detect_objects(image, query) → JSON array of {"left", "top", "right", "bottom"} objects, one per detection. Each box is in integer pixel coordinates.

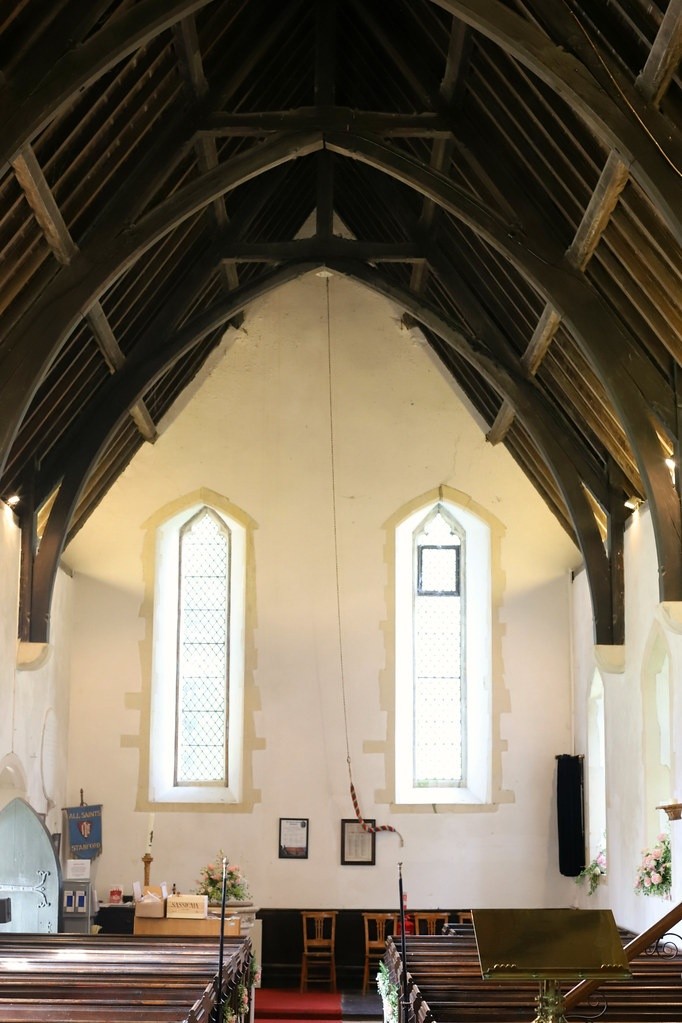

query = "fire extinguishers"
[{"left": 396, "top": 912, "right": 415, "bottom": 935}]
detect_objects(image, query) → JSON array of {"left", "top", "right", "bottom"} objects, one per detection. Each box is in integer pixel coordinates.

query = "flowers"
[
  {"left": 246, "top": 949, "right": 261, "bottom": 988},
  {"left": 234, "top": 982, "right": 250, "bottom": 1012},
  {"left": 189, "top": 849, "right": 253, "bottom": 903},
  {"left": 633, "top": 833, "right": 671, "bottom": 902},
  {"left": 574, "top": 830, "right": 607, "bottom": 897},
  {"left": 375, "top": 960, "right": 399, "bottom": 1023},
  {"left": 221, "top": 993, "right": 238, "bottom": 1023}
]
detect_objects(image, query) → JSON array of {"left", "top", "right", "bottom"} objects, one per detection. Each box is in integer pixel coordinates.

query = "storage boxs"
[
  {"left": 166, "top": 894, "right": 208, "bottom": 919},
  {"left": 132, "top": 881, "right": 171, "bottom": 918}
]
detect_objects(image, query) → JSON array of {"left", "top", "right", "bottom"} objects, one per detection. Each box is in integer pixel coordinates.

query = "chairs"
[
  {"left": 413, "top": 913, "right": 450, "bottom": 935},
  {"left": 362, "top": 913, "right": 401, "bottom": 997},
  {"left": 300, "top": 911, "right": 338, "bottom": 996}
]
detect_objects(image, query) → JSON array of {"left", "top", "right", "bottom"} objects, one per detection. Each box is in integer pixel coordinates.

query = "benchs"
[
  {"left": 382, "top": 930, "right": 682, "bottom": 1023},
  {"left": 0, "top": 933, "right": 252, "bottom": 1023}
]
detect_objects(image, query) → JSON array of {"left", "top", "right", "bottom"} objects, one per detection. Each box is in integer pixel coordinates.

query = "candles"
[{"left": 145, "top": 813, "right": 155, "bottom": 854}]
